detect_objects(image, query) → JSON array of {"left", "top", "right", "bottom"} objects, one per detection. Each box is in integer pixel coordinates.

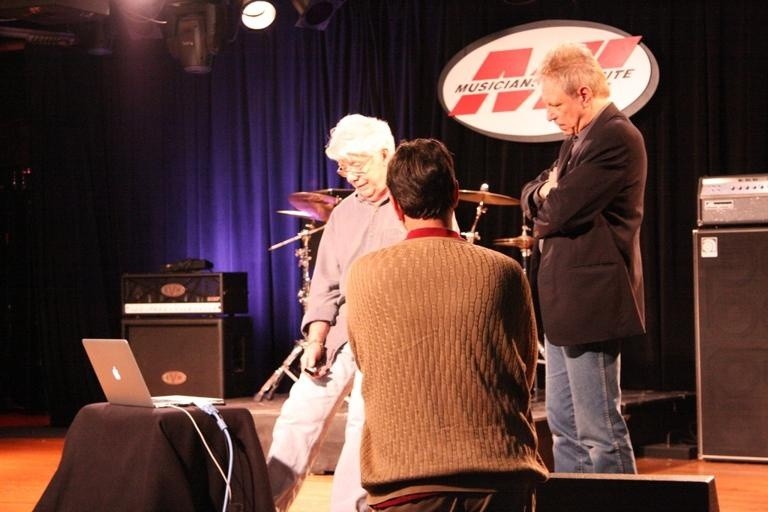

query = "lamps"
[
  {"left": 238, "top": 0, "right": 278, "bottom": 31},
  {"left": 176, "top": 15, "right": 214, "bottom": 76}
]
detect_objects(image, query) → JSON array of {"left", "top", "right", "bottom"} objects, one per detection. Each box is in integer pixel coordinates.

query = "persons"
[
  {"left": 266, "top": 114, "right": 460, "bottom": 511},
  {"left": 344, "top": 138, "right": 550, "bottom": 512},
  {"left": 519, "top": 44, "right": 647, "bottom": 475}
]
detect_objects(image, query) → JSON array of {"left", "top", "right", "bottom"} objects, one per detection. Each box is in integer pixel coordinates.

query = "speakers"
[
  {"left": 691, "top": 225, "right": 768, "bottom": 463},
  {"left": 120, "top": 315, "right": 254, "bottom": 398}
]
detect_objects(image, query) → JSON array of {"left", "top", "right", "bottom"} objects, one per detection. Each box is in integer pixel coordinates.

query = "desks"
[{"left": 30, "top": 403, "right": 277, "bottom": 511}]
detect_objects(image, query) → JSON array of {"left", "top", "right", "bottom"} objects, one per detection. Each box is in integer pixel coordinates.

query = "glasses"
[{"left": 336, "top": 158, "right": 371, "bottom": 177}]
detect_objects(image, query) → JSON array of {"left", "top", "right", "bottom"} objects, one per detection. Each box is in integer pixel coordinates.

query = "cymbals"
[
  {"left": 277, "top": 210, "right": 315, "bottom": 220},
  {"left": 458, "top": 189, "right": 520, "bottom": 206},
  {"left": 288, "top": 192, "right": 343, "bottom": 223},
  {"left": 315, "top": 189, "right": 355, "bottom": 194},
  {"left": 268, "top": 226, "right": 326, "bottom": 250},
  {"left": 494, "top": 236, "right": 537, "bottom": 249}
]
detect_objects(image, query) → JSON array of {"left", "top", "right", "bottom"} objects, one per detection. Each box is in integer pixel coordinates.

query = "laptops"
[{"left": 82, "top": 338, "right": 225, "bottom": 408}]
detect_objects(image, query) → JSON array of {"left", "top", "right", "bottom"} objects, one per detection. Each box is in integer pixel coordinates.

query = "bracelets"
[{"left": 303, "top": 338, "right": 325, "bottom": 347}]
